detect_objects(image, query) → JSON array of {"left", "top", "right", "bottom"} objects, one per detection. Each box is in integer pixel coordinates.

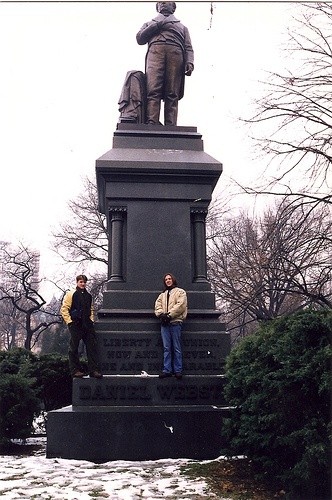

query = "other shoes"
[
  {"left": 159, "top": 373, "right": 172, "bottom": 378},
  {"left": 92, "top": 368, "right": 103, "bottom": 378},
  {"left": 175, "top": 372, "right": 182, "bottom": 379},
  {"left": 71, "top": 370, "right": 85, "bottom": 378}
]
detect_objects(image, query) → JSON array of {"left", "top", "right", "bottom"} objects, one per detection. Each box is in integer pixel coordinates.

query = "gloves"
[{"left": 159, "top": 312, "right": 172, "bottom": 328}]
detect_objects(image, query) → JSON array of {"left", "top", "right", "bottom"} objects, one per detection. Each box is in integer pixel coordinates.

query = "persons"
[
  {"left": 60, "top": 275, "right": 103, "bottom": 379},
  {"left": 136, "top": 2, "right": 195, "bottom": 126},
  {"left": 154, "top": 273, "right": 188, "bottom": 379}
]
